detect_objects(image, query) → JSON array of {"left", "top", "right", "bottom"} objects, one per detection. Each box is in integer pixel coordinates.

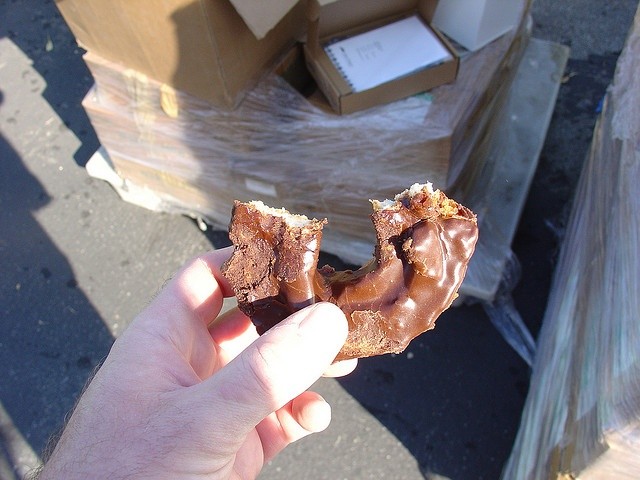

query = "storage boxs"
[
  {"left": 432, "top": 1, "right": 526, "bottom": 53},
  {"left": 51, "top": 0, "right": 314, "bottom": 113},
  {"left": 301, "top": 0, "right": 463, "bottom": 118}
]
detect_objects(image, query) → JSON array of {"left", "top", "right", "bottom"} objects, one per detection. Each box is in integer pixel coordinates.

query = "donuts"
[{"left": 222, "top": 181, "right": 479, "bottom": 364}]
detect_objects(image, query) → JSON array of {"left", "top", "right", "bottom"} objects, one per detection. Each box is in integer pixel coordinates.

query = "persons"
[{"left": 24, "top": 243, "right": 361, "bottom": 480}]
